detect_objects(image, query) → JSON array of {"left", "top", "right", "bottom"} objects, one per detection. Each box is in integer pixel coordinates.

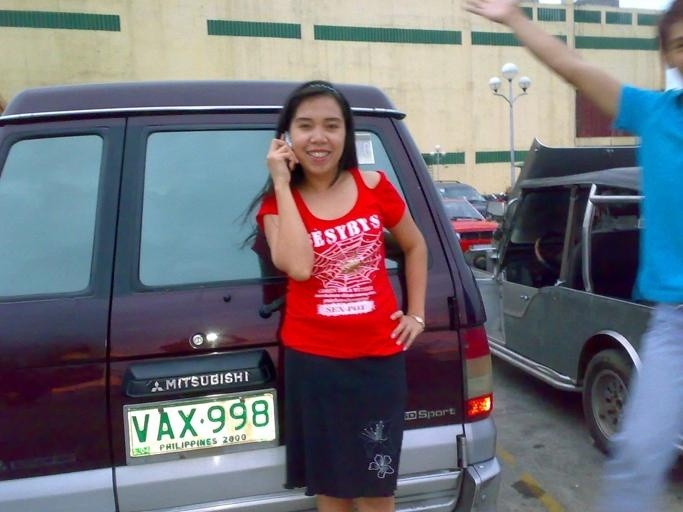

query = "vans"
[{"left": 1, "top": 79, "right": 501, "bottom": 512}]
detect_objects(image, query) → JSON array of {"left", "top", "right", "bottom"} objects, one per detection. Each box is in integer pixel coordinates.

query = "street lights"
[{"left": 491, "top": 62, "right": 531, "bottom": 184}]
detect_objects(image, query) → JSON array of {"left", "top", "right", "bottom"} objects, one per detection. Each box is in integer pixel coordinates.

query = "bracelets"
[{"left": 405, "top": 314, "right": 427, "bottom": 330}]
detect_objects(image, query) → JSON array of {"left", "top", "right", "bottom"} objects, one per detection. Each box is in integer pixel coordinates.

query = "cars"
[
  {"left": 433, "top": 179, "right": 500, "bottom": 264},
  {"left": 464, "top": 139, "right": 683, "bottom": 458}
]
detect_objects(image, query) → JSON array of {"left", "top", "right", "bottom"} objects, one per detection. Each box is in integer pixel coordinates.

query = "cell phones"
[{"left": 284, "top": 131, "right": 293, "bottom": 148}]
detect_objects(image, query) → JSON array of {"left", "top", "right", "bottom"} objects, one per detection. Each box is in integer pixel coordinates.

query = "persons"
[
  {"left": 459, "top": 0, "right": 682, "bottom": 512},
  {"left": 234, "top": 80, "right": 430, "bottom": 511}
]
